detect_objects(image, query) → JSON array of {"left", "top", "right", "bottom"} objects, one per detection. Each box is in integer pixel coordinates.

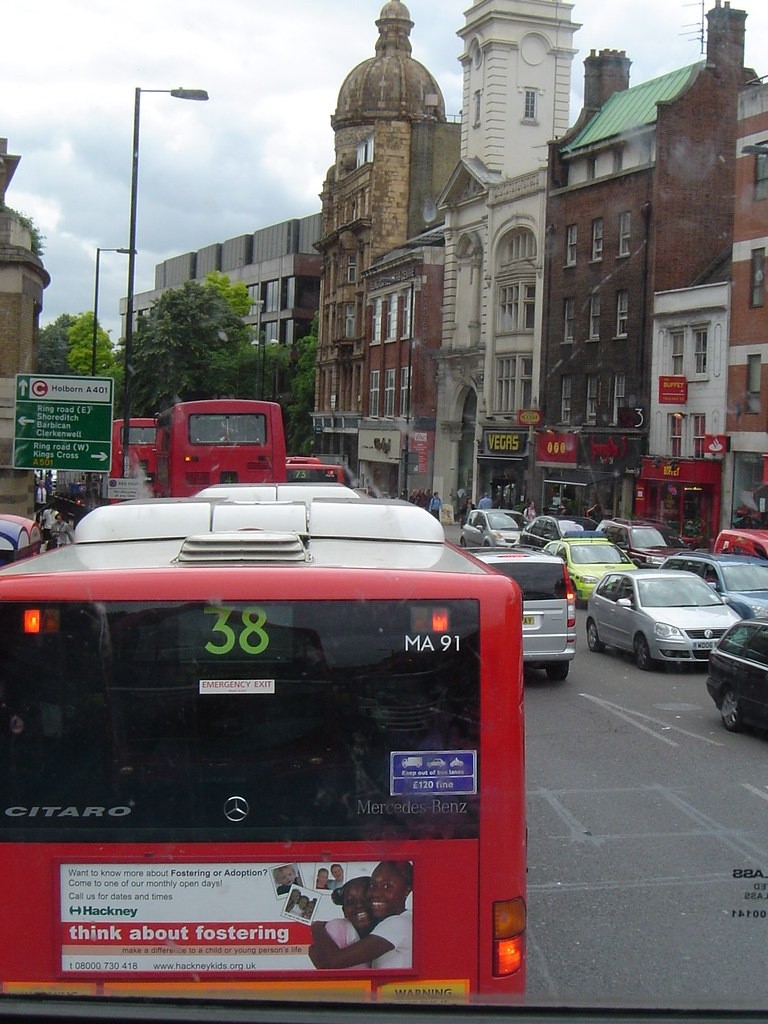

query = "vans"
[{"left": 462, "top": 543, "right": 577, "bottom": 680}]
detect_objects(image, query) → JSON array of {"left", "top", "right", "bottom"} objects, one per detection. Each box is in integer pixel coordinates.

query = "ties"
[{"left": 41, "top": 487, "right": 43, "bottom": 502}]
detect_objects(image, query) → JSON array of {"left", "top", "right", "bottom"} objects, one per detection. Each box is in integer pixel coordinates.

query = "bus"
[
  {"left": 0, "top": 484, "right": 528, "bottom": 1011},
  {"left": 159, "top": 400, "right": 288, "bottom": 499},
  {"left": 285, "top": 456, "right": 345, "bottom": 484},
  {"left": 98, "top": 417, "right": 159, "bottom": 506}
]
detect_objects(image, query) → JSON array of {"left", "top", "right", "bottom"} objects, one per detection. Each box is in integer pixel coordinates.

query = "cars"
[
  {"left": 585, "top": 569, "right": 748, "bottom": 671},
  {"left": 519, "top": 514, "right": 600, "bottom": 551},
  {"left": 713, "top": 529, "right": 768, "bottom": 560},
  {"left": 705, "top": 616, "right": 768, "bottom": 734},
  {"left": 459, "top": 508, "right": 529, "bottom": 548},
  {"left": 540, "top": 531, "right": 639, "bottom": 610}
]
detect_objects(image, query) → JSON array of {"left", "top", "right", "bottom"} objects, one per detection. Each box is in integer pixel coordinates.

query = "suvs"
[
  {"left": 594, "top": 516, "right": 691, "bottom": 571},
  {"left": 655, "top": 550, "right": 768, "bottom": 622}
]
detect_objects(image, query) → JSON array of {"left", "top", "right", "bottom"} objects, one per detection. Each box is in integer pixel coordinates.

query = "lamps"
[
  {"left": 546, "top": 429, "right": 558, "bottom": 435},
  {"left": 533, "top": 429, "right": 541, "bottom": 435},
  {"left": 651, "top": 458, "right": 660, "bottom": 468},
  {"left": 668, "top": 459, "right": 679, "bottom": 470},
  {"left": 504, "top": 416, "right": 513, "bottom": 421},
  {"left": 487, "top": 417, "right": 496, "bottom": 422}
]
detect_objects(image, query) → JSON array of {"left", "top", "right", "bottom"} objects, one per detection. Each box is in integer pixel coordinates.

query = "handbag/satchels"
[
  {"left": 41, "top": 519, "right": 46, "bottom": 526},
  {"left": 47, "top": 541, "right": 58, "bottom": 552}
]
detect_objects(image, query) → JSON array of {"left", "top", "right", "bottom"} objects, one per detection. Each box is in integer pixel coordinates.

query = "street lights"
[
  {"left": 121, "top": 85, "right": 211, "bottom": 479},
  {"left": 90, "top": 248, "right": 139, "bottom": 378},
  {"left": 378, "top": 276, "right": 415, "bottom": 500}
]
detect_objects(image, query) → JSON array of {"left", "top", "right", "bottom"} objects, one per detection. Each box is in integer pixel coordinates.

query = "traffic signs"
[
  {"left": 14, "top": 399, "right": 113, "bottom": 443},
  {"left": 15, "top": 372, "right": 114, "bottom": 404},
  {"left": 12, "top": 438, "right": 111, "bottom": 473}
]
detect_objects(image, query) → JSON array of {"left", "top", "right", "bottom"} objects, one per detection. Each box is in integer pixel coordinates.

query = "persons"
[
  {"left": 325, "top": 875, "right": 378, "bottom": 969},
  {"left": 328, "top": 864, "right": 344, "bottom": 889},
  {"left": 50, "top": 512, "right": 73, "bottom": 546},
  {"left": 307, "top": 860, "right": 413, "bottom": 969},
  {"left": 273, "top": 864, "right": 303, "bottom": 895},
  {"left": 46, "top": 469, "right": 53, "bottom": 492},
  {"left": 586, "top": 493, "right": 604, "bottom": 523},
  {"left": 303, "top": 898, "right": 317, "bottom": 919},
  {"left": 478, "top": 492, "right": 493, "bottom": 509},
  {"left": 522, "top": 497, "right": 537, "bottom": 522},
  {"left": 491, "top": 491, "right": 515, "bottom": 510},
  {"left": 316, "top": 868, "right": 330, "bottom": 890},
  {"left": 399, "top": 491, "right": 407, "bottom": 500},
  {"left": 290, "top": 895, "right": 309, "bottom": 917},
  {"left": 558, "top": 497, "right": 575, "bottom": 516},
  {"left": 409, "top": 487, "right": 441, "bottom": 521},
  {"left": 34, "top": 480, "right": 52, "bottom": 510},
  {"left": 458, "top": 495, "right": 478, "bottom": 546},
  {"left": 41, "top": 503, "right": 59, "bottom": 541},
  {"left": 286, "top": 890, "right": 301, "bottom": 912}
]
